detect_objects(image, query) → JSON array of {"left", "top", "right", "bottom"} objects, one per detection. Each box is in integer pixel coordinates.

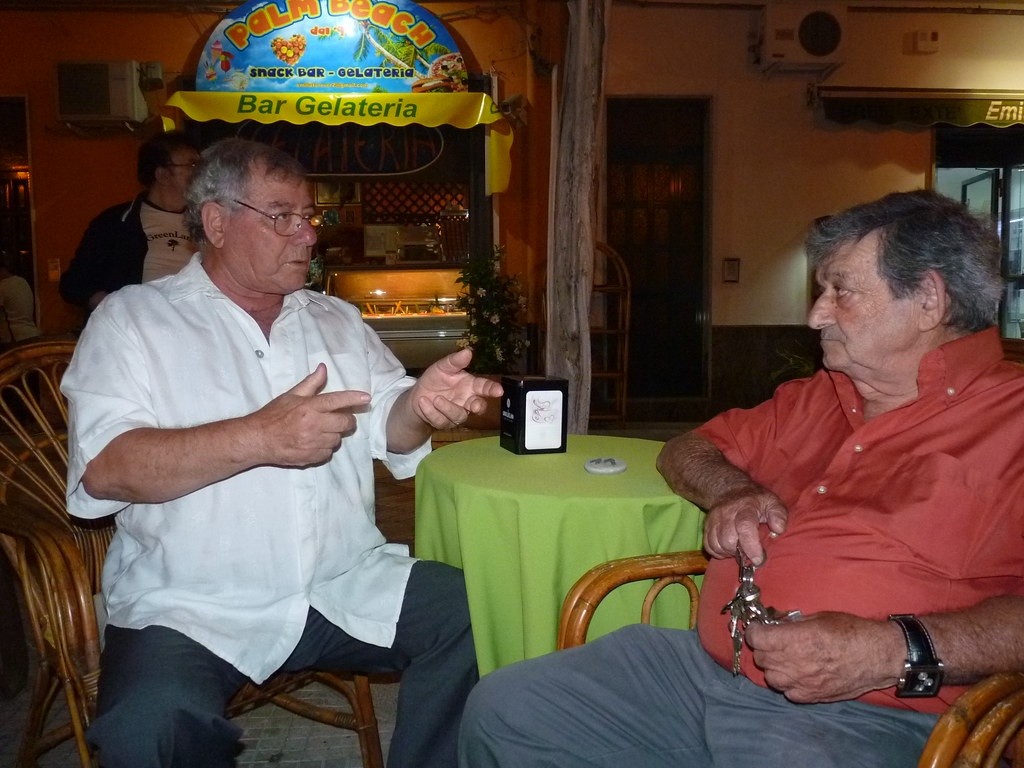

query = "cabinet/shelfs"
[
  {"left": 540, "top": 246, "right": 630, "bottom": 429},
  {"left": 328, "top": 265, "right": 471, "bottom": 370}
]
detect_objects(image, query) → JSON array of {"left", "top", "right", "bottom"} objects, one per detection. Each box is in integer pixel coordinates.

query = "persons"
[
  {"left": 456, "top": 191, "right": 1023, "bottom": 768},
  {"left": 59, "top": 140, "right": 477, "bottom": 768},
  {"left": 59, "top": 132, "right": 210, "bottom": 314},
  {"left": 0, "top": 266, "right": 41, "bottom": 342}
]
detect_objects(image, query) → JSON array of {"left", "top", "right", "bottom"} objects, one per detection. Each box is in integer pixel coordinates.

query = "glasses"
[
  {"left": 231, "top": 198, "right": 326, "bottom": 238},
  {"left": 166, "top": 159, "right": 205, "bottom": 172}
]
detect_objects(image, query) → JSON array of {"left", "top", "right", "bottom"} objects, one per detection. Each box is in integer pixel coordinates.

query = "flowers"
[{"left": 451, "top": 244, "right": 533, "bottom": 372}]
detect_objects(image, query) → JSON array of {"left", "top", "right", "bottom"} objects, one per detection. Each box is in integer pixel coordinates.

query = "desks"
[{"left": 411, "top": 429, "right": 712, "bottom": 676}]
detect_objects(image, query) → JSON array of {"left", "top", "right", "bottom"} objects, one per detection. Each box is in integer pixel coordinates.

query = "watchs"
[{"left": 887, "top": 614, "right": 944, "bottom": 697}]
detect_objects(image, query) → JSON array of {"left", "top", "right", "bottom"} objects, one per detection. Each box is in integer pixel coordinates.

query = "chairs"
[
  {"left": 0, "top": 335, "right": 390, "bottom": 768},
  {"left": 552, "top": 337, "right": 1024, "bottom": 767}
]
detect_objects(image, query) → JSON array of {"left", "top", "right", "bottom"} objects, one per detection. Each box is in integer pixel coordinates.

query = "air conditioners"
[
  {"left": 756, "top": 1, "right": 848, "bottom": 73},
  {"left": 54, "top": 58, "right": 149, "bottom": 130}
]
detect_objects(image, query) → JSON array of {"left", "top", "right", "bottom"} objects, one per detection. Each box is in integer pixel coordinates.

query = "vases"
[{"left": 463, "top": 371, "right": 505, "bottom": 428}]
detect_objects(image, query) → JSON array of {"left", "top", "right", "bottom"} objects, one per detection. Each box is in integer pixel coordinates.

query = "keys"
[{"left": 721, "top": 543, "right": 802, "bottom": 679}]
